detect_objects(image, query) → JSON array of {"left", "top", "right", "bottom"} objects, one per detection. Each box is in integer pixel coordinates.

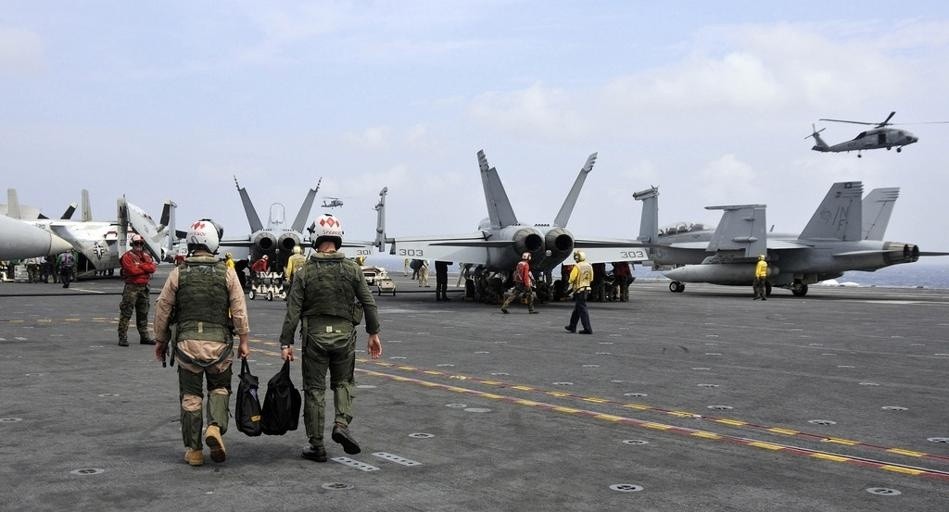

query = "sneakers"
[
  {"left": 119, "top": 337, "right": 129, "bottom": 346},
  {"left": 184, "top": 450, "right": 204, "bottom": 465},
  {"left": 579, "top": 330, "right": 592, "bottom": 334},
  {"left": 501, "top": 308, "right": 508, "bottom": 313},
  {"left": 565, "top": 326, "right": 575, "bottom": 332},
  {"left": 140, "top": 337, "right": 156, "bottom": 345},
  {"left": 205, "top": 425, "right": 226, "bottom": 462},
  {"left": 529, "top": 310, "right": 539, "bottom": 314}
]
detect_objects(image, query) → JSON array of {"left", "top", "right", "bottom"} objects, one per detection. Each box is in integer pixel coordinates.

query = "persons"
[
  {"left": 403, "top": 257, "right": 453, "bottom": 301},
  {"left": 4, "top": 248, "right": 78, "bottom": 288},
  {"left": 152, "top": 219, "right": 251, "bottom": 465},
  {"left": 752, "top": 254, "right": 769, "bottom": 301},
  {"left": 500, "top": 252, "right": 539, "bottom": 314},
  {"left": 560, "top": 262, "right": 632, "bottom": 301},
  {"left": 563, "top": 249, "right": 593, "bottom": 334},
  {"left": 224, "top": 245, "right": 307, "bottom": 300},
  {"left": 118, "top": 234, "right": 157, "bottom": 346},
  {"left": 279, "top": 213, "right": 383, "bottom": 462}
]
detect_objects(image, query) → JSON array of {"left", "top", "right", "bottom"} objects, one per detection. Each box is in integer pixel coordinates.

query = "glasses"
[{"left": 135, "top": 241, "right": 144, "bottom": 246}]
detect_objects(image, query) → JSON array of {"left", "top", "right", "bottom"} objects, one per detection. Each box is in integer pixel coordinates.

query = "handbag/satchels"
[
  {"left": 235, "top": 357, "right": 261, "bottom": 436},
  {"left": 261, "top": 357, "right": 301, "bottom": 435}
]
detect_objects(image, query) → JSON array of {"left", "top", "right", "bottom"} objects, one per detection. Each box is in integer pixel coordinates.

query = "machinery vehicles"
[
  {"left": 249, "top": 272, "right": 286, "bottom": 301},
  {"left": 374, "top": 271, "right": 397, "bottom": 296}
]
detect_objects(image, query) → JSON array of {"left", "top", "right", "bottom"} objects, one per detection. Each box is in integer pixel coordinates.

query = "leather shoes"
[
  {"left": 301, "top": 446, "right": 327, "bottom": 462},
  {"left": 332, "top": 425, "right": 361, "bottom": 454}
]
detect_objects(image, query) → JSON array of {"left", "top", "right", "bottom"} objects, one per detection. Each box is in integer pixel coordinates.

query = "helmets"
[
  {"left": 574, "top": 251, "right": 585, "bottom": 261},
  {"left": 758, "top": 255, "right": 765, "bottom": 260},
  {"left": 225, "top": 252, "right": 231, "bottom": 258},
  {"left": 294, "top": 246, "right": 301, "bottom": 254},
  {"left": 186, "top": 218, "right": 223, "bottom": 253},
  {"left": 307, "top": 213, "right": 344, "bottom": 249},
  {"left": 522, "top": 252, "right": 531, "bottom": 260}
]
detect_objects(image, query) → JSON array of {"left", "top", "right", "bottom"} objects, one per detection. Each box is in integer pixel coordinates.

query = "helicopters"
[{"left": 805, "top": 110, "right": 920, "bottom": 159}]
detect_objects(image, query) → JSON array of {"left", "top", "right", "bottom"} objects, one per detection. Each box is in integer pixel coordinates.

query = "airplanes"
[
  {"left": 1, "top": 189, "right": 179, "bottom": 280},
  {"left": 213, "top": 173, "right": 378, "bottom": 275},
  {"left": 632, "top": 180, "right": 948, "bottom": 298},
  {"left": 367, "top": 147, "right": 660, "bottom": 299}
]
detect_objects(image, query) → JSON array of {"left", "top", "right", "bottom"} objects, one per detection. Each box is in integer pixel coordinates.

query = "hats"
[{"left": 131, "top": 234, "right": 143, "bottom": 240}]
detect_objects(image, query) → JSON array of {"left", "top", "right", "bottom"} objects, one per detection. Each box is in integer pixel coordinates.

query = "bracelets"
[{"left": 280, "top": 345, "right": 290, "bottom": 350}]
